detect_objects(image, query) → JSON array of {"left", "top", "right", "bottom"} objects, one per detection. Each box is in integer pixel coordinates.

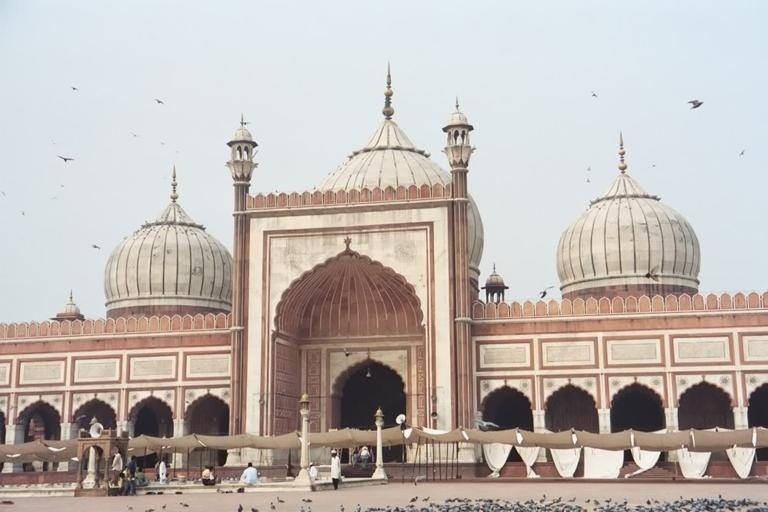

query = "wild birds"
[
  {"left": 590, "top": 89, "right": 599, "bottom": 99},
  {"left": 238, "top": 504, "right": 260, "bottom": 511},
  {"left": 537, "top": 286, "right": 555, "bottom": 298},
  {"left": 644, "top": 264, "right": 664, "bottom": 283},
  {"left": 737, "top": 148, "right": 747, "bottom": 157},
  {"left": 124, "top": 503, "right": 189, "bottom": 512},
  {"left": 356, "top": 503, "right": 362, "bottom": 511},
  {"left": 301, "top": 497, "right": 312, "bottom": 511},
  {"left": 0, "top": 85, "right": 181, "bottom": 259},
  {"left": 367, "top": 494, "right": 767, "bottom": 511},
  {"left": 339, "top": 504, "right": 345, "bottom": 511}
]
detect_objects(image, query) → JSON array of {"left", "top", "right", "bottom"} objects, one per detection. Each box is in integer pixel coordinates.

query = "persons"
[
  {"left": 110, "top": 445, "right": 123, "bottom": 486},
  {"left": 119, "top": 467, "right": 135, "bottom": 480},
  {"left": 153, "top": 457, "right": 162, "bottom": 481},
  {"left": 331, "top": 449, "right": 341, "bottom": 490},
  {"left": 309, "top": 461, "right": 318, "bottom": 478},
  {"left": 136, "top": 467, "right": 145, "bottom": 484},
  {"left": 122, "top": 454, "right": 136, "bottom": 496},
  {"left": 202, "top": 464, "right": 210, "bottom": 479},
  {"left": 352, "top": 446, "right": 360, "bottom": 468},
  {"left": 159, "top": 457, "right": 167, "bottom": 484},
  {"left": 208, "top": 465, "right": 217, "bottom": 480},
  {"left": 240, "top": 462, "right": 258, "bottom": 484},
  {"left": 360, "top": 444, "right": 370, "bottom": 469}
]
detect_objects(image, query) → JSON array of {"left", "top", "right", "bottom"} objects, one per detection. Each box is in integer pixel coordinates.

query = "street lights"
[
  {"left": 299, "top": 393, "right": 311, "bottom": 467},
  {"left": 374, "top": 408, "right": 384, "bottom": 468}
]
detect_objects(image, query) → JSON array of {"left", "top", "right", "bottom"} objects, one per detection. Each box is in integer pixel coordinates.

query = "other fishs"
[
  {"left": 276, "top": 496, "right": 286, "bottom": 509},
  {"left": 269, "top": 502, "right": 276, "bottom": 510}
]
